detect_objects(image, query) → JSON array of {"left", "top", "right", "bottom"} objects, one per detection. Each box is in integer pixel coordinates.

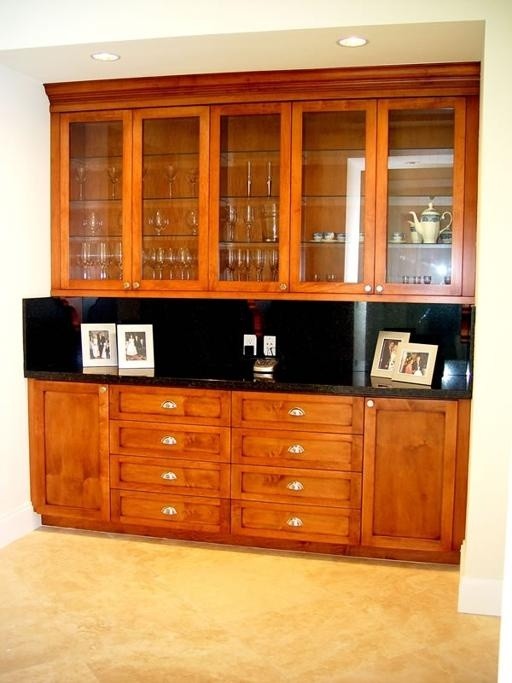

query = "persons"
[
  {"left": 381, "top": 341, "right": 424, "bottom": 376},
  {"left": 90, "top": 333, "right": 146, "bottom": 359}
]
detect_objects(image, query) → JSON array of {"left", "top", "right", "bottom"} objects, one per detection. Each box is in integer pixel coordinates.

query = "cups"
[
  {"left": 386, "top": 220, "right": 452, "bottom": 243},
  {"left": 310, "top": 230, "right": 364, "bottom": 243}
]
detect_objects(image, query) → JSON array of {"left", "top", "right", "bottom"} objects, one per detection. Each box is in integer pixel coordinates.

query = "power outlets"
[{"left": 243, "top": 334, "right": 278, "bottom": 356}]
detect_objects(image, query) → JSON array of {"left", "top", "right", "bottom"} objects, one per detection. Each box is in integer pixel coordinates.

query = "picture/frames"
[
  {"left": 79, "top": 321, "right": 156, "bottom": 369},
  {"left": 369, "top": 329, "right": 441, "bottom": 387}
]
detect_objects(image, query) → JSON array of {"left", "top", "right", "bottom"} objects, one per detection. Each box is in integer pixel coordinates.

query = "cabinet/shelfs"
[
  {"left": 27, "top": 378, "right": 109, "bottom": 531},
  {"left": 364, "top": 392, "right": 469, "bottom": 566},
  {"left": 112, "top": 383, "right": 362, "bottom": 558},
  {"left": 50, "top": 95, "right": 480, "bottom": 304}
]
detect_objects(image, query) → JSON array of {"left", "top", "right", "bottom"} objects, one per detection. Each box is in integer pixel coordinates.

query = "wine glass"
[{"left": 72, "top": 158, "right": 279, "bottom": 282}]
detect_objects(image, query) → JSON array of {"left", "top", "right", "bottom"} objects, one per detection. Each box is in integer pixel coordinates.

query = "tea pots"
[{"left": 409, "top": 201, "right": 453, "bottom": 243}]
